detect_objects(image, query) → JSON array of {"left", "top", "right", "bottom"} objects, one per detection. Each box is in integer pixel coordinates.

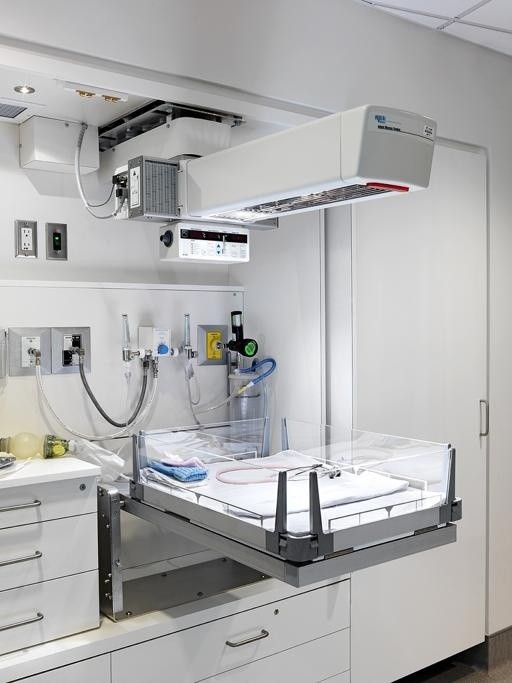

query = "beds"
[{"left": 118, "top": 413, "right": 464, "bottom": 588}]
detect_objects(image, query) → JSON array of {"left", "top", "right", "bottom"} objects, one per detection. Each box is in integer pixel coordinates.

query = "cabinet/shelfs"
[
  {"left": 1, "top": 519, "right": 358, "bottom": 683},
  {"left": 1, "top": 454, "right": 102, "bottom": 657}
]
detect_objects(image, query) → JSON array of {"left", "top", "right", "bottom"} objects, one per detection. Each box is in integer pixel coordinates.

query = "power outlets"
[
  {"left": 13, "top": 218, "right": 39, "bottom": 257},
  {"left": 20, "top": 335, "right": 42, "bottom": 369}
]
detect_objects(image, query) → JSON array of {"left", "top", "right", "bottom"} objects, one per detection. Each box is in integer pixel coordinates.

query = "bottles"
[{"left": 1, "top": 432, "right": 75, "bottom": 461}]
